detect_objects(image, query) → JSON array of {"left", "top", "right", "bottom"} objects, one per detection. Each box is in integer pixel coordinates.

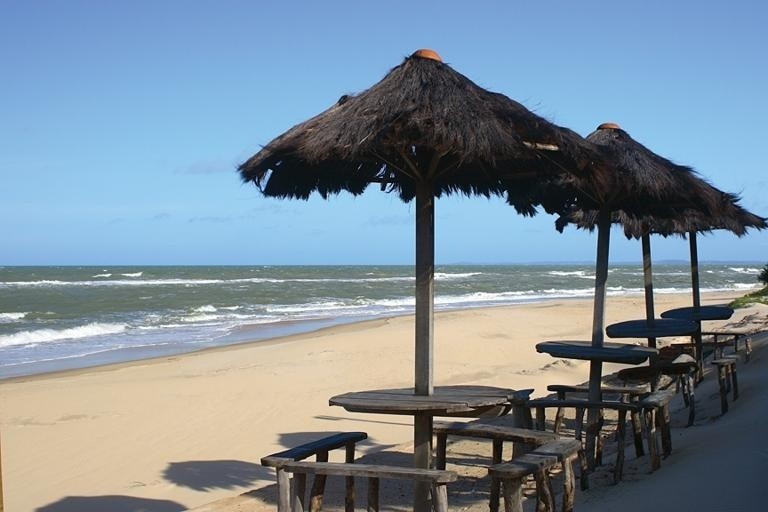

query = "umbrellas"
[
  {"left": 236, "top": 46, "right": 638, "bottom": 511},
  {"left": 548, "top": 121, "right": 716, "bottom": 472},
  {"left": 622, "top": 203, "right": 768, "bottom": 380},
  {"left": 552, "top": 177, "right": 747, "bottom": 394}
]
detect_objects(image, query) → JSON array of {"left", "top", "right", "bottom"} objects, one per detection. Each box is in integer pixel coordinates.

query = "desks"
[
  {"left": 606, "top": 306, "right": 734, "bottom": 391},
  {"left": 327, "top": 385, "right": 514, "bottom": 512}
]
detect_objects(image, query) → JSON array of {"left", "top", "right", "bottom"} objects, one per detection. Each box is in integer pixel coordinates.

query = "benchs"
[
  {"left": 487, "top": 439, "right": 582, "bottom": 512},
  {"left": 260, "top": 431, "right": 368, "bottom": 512},
  {"left": 432, "top": 419, "right": 563, "bottom": 512},
  {"left": 282, "top": 460, "right": 457, "bottom": 512},
  {"left": 516, "top": 330, "right": 750, "bottom": 489}
]
